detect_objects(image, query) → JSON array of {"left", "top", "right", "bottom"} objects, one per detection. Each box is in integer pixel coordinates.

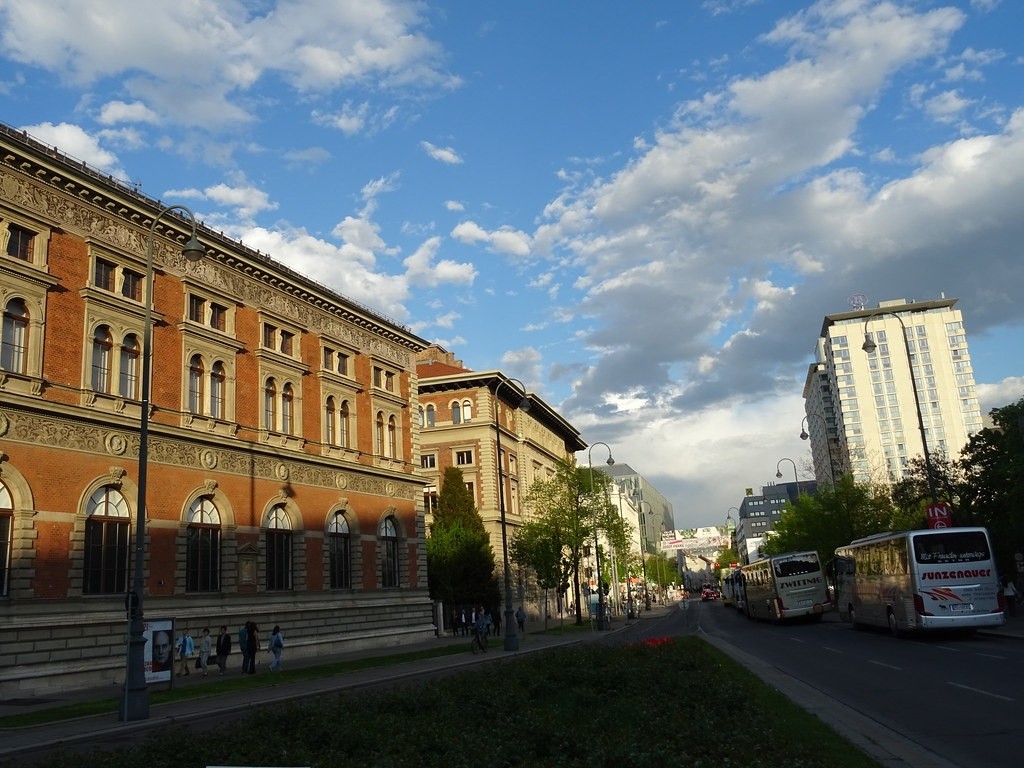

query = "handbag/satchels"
[
  {"left": 195, "top": 655, "right": 202, "bottom": 668},
  {"left": 274, "top": 635, "right": 282, "bottom": 648}
]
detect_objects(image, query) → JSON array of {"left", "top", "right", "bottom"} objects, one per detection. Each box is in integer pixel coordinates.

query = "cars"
[{"left": 701, "top": 589, "right": 717, "bottom": 600}]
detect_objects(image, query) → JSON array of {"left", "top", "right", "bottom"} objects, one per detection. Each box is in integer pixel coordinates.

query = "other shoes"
[
  {"left": 183, "top": 673, "right": 189, "bottom": 676},
  {"left": 219, "top": 673, "right": 223, "bottom": 675},
  {"left": 270, "top": 666, "right": 272, "bottom": 672},
  {"left": 203, "top": 672, "right": 208, "bottom": 676}
]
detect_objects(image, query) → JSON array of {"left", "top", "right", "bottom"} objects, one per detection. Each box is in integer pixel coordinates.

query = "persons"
[
  {"left": 199, "top": 629, "right": 211, "bottom": 676},
  {"left": 451, "top": 606, "right": 502, "bottom": 646},
  {"left": 175, "top": 628, "right": 194, "bottom": 677},
  {"left": 515, "top": 606, "right": 526, "bottom": 632},
  {"left": 152, "top": 630, "right": 172, "bottom": 672},
  {"left": 267, "top": 625, "right": 283, "bottom": 672},
  {"left": 570, "top": 601, "right": 574, "bottom": 614},
  {"left": 216, "top": 626, "right": 231, "bottom": 676},
  {"left": 239, "top": 622, "right": 260, "bottom": 674}
]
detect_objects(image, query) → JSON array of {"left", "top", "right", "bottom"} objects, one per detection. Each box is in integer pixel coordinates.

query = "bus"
[
  {"left": 826, "top": 527, "right": 1008, "bottom": 639},
  {"left": 723, "top": 550, "right": 833, "bottom": 622}
]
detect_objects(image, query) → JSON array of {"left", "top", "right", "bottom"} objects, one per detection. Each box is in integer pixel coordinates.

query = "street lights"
[
  {"left": 495, "top": 378, "right": 531, "bottom": 651},
  {"left": 589, "top": 442, "right": 614, "bottom": 630},
  {"left": 862, "top": 310, "right": 938, "bottom": 504},
  {"left": 618, "top": 479, "right": 639, "bottom": 619},
  {"left": 637, "top": 500, "right": 653, "bottom": 610},
  {"left": 799, "top": 414, "right": 846, "bottom": 548},
  {"left": 652, "top": 514, "right": 666, "bottom": 605},
  {"left": 725, "top": 507, "right": 747, "bottom": 565},
  {"left": 776, "top": 458, "right": 801, "bottom": 497},
  {"left": 120, "top": 206, "right": 206, "bottom": 721}
]
